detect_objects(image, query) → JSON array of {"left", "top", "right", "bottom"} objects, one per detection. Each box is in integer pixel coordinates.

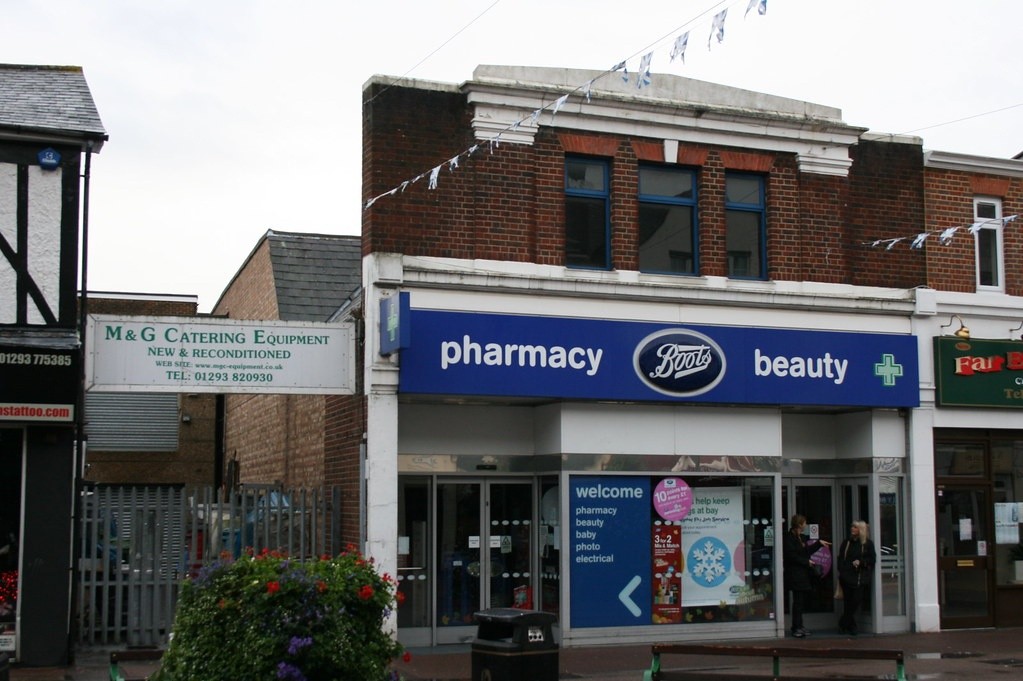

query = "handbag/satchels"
[{"left": 833, "top": 574, "right": 845, "bottom": 602}]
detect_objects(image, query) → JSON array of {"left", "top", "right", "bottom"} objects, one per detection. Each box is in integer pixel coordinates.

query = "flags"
[
  {"left": 366, "top": 0, "right": 769, "bottom": 209},
  {"left": 886, "top": 214, "right": 1016, "bottom": 250}
]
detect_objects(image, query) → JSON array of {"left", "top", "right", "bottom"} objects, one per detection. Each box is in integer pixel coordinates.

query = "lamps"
[
  {"left": 942, "top": 314, "right": 970, "bottom": 340},
  {"left": 183, "top": 416, "right": 190, "bottom": 422}
]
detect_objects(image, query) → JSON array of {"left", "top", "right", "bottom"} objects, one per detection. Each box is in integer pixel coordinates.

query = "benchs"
[{"left": 644, "top": 642, "right": 907, "bottom": 681}]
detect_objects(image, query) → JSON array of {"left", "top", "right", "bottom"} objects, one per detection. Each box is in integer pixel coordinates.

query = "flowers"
[{"left": 145, "top": 544, "right": 413, "bottom": 681}]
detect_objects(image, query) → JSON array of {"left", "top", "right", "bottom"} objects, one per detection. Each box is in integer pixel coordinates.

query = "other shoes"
[
  {"left": 848, "top": 624, "right": 858, "bottom": 634},
  {"left": 791, "top": 627, "right": 811, "bottom": 638},
  {"left": 838, "top": 626, "right": 845, "bottom": 634}
]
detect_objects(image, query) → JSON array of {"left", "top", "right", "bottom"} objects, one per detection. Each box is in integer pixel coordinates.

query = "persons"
[
  {"left": 783, "top": 515, "right": 833, "bottom": 638},
  {"left": 836, "top": 519, "right": 876, "bottom": 636}
]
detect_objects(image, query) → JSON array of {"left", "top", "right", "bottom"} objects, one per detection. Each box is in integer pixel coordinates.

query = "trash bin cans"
[{"left": 470, "top": 607, "right": 561, "bottom": 681}]
